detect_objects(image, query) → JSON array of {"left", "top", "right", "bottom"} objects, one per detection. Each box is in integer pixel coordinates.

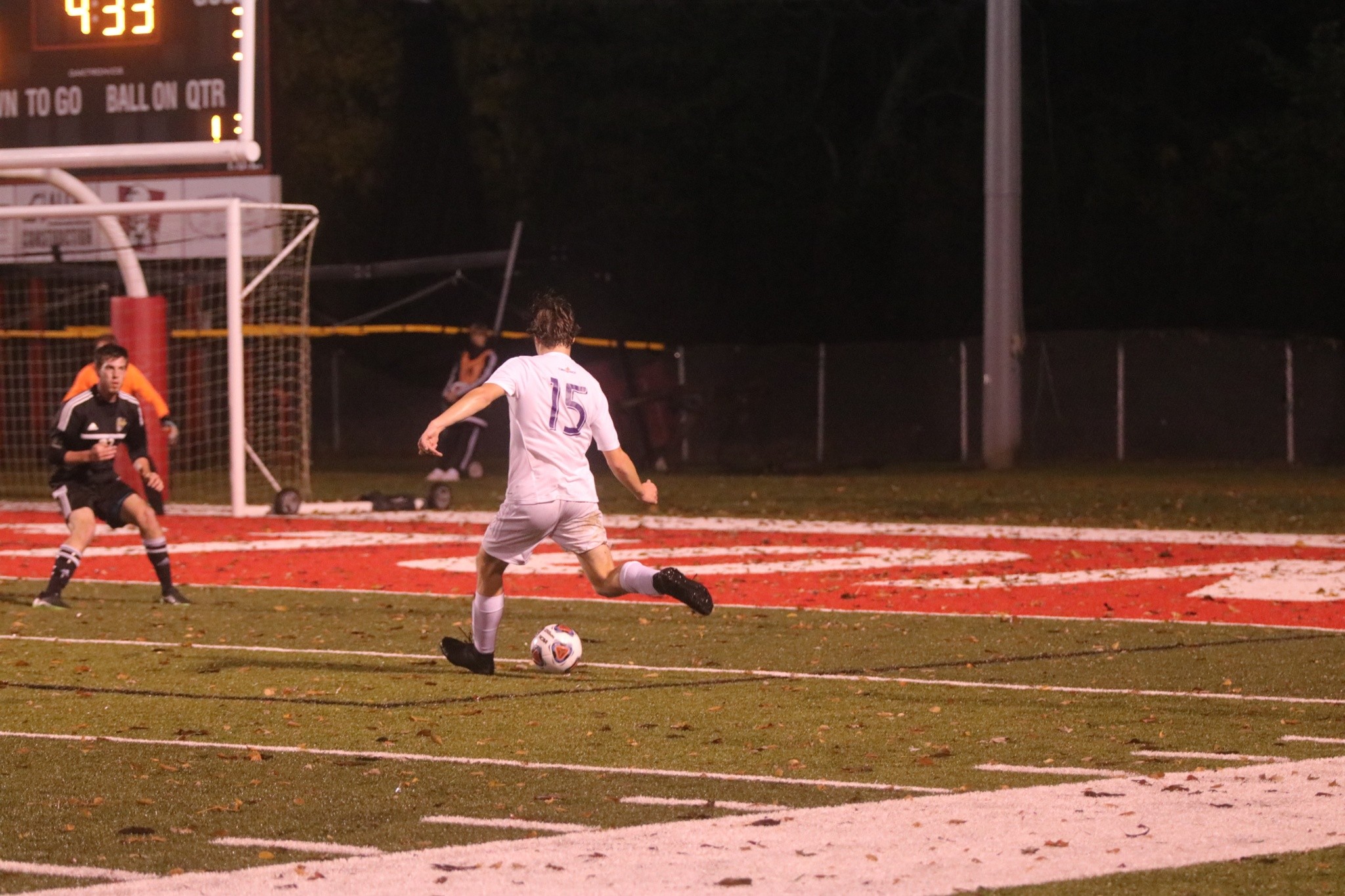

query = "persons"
[
  {"left": 29, "top": 333, "right": 191, "bottom": 607},
  {"left": 427, "top": 320, "right": 497, "bottom": 482},
  {"left": 418, "top": 292, "right": 713, "bottom": 677}
]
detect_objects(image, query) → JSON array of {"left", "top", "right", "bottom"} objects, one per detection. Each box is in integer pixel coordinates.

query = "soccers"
[{"left": 531, "top": 623, "right": 583, "bottom": 673}]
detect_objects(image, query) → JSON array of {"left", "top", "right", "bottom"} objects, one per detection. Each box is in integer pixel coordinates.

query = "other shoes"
[
  {"left": 425, "top": 467, "right": 445, "bottom": 482},
  {"left": 441, "top": 468, "right": 459, "bottom": 482}
]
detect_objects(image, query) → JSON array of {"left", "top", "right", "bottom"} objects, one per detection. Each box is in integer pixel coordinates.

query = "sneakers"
[
  {"left": 653, "top": 567, "right": 714, "bottom": 616},
  {"left": 31, "top": 592, "right": 72, "bottom": 610},
  {"left": 161, "top": 589, "right": 192, "bottom": 606},
  {"left": 439, "top": 627, "right": 495, "bottom": 676}
]
